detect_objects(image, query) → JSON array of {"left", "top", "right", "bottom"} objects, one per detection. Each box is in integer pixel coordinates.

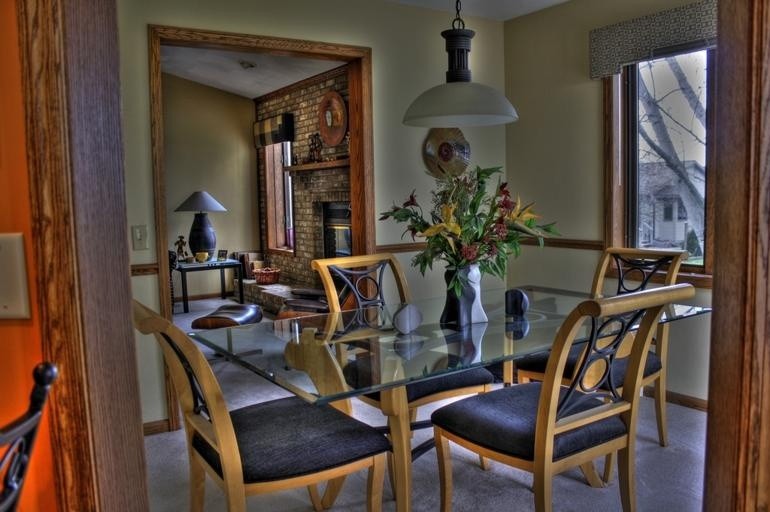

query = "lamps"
[
  {"left": 402, "top": 1, "right": 519, "bottom": 130},
  {"left": 174, "top": 190, "right": 228, "bottom": 261}
]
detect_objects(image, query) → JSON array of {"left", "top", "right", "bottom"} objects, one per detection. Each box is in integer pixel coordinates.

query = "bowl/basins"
[{"left": 194, "top": 252, "right": 209, "bottom": 263}]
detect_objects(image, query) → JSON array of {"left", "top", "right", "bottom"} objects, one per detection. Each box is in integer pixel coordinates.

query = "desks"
[{"left": 170, "top": 258, "right": 244, "bottom": 313}]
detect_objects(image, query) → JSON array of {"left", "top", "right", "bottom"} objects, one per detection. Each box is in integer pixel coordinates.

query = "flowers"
[{"left": 376, "top": 164, "right": 564, "bottom": 298}]
